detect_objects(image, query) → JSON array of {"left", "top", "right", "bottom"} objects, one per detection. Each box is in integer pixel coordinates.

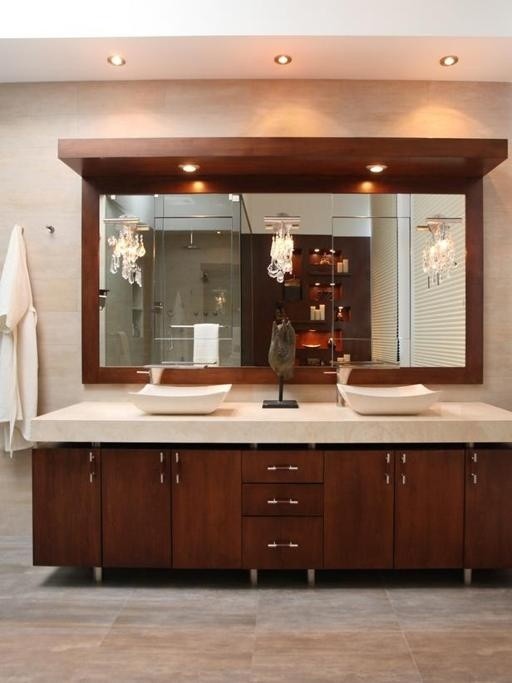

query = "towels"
[{"left": 194, "top": 324, "right": 219, "bottom": 366}]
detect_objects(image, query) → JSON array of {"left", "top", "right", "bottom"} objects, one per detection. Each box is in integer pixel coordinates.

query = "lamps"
[
  {"left": 103, "top": 218, "right": 150, "bottom": 287},
  {"left": 416, "top": 216, "right": 463, "bottom": 282},
  {"left": 263, "top": 216, "right": 302, "bottom": 283}
]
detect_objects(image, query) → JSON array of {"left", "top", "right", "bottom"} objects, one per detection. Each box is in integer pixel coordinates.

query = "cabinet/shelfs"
[
  {"left": 102, "top": 449, "right": 242, "bottom": 568},
  {"left": 325, "top": 451, "right": 465, "bottom": 569},
  {"left": 242, "top": 450, "right": 324, "bottom": 586},
  {"left": 242, "top": 234, "right": 371, "bottom": 365},
  {"left": 23, "top": 448, "right": 102, "bottom": 581},
  {"left": 465, "top": 449, "right": 512, "bottom": 584}
]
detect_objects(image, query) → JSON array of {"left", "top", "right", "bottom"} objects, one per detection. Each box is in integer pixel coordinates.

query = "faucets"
[
  {"left": 144, "top": 364, "right": 208, "bottom": 385},
  {"left": 333, "top": 359, "right": 400, "bottom": 384}
]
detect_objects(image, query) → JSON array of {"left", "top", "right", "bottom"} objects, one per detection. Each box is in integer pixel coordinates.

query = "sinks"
[
  {"left": 337, "top": 384, "right": 442, "bottom": 416},
  {"left": 127, "top": 384, "right": 232, "bottom": 415}
]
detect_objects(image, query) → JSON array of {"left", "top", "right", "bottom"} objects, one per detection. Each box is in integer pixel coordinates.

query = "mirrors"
[{"left": 82, "top": 176, "right": 484, "bottom": 384}]
